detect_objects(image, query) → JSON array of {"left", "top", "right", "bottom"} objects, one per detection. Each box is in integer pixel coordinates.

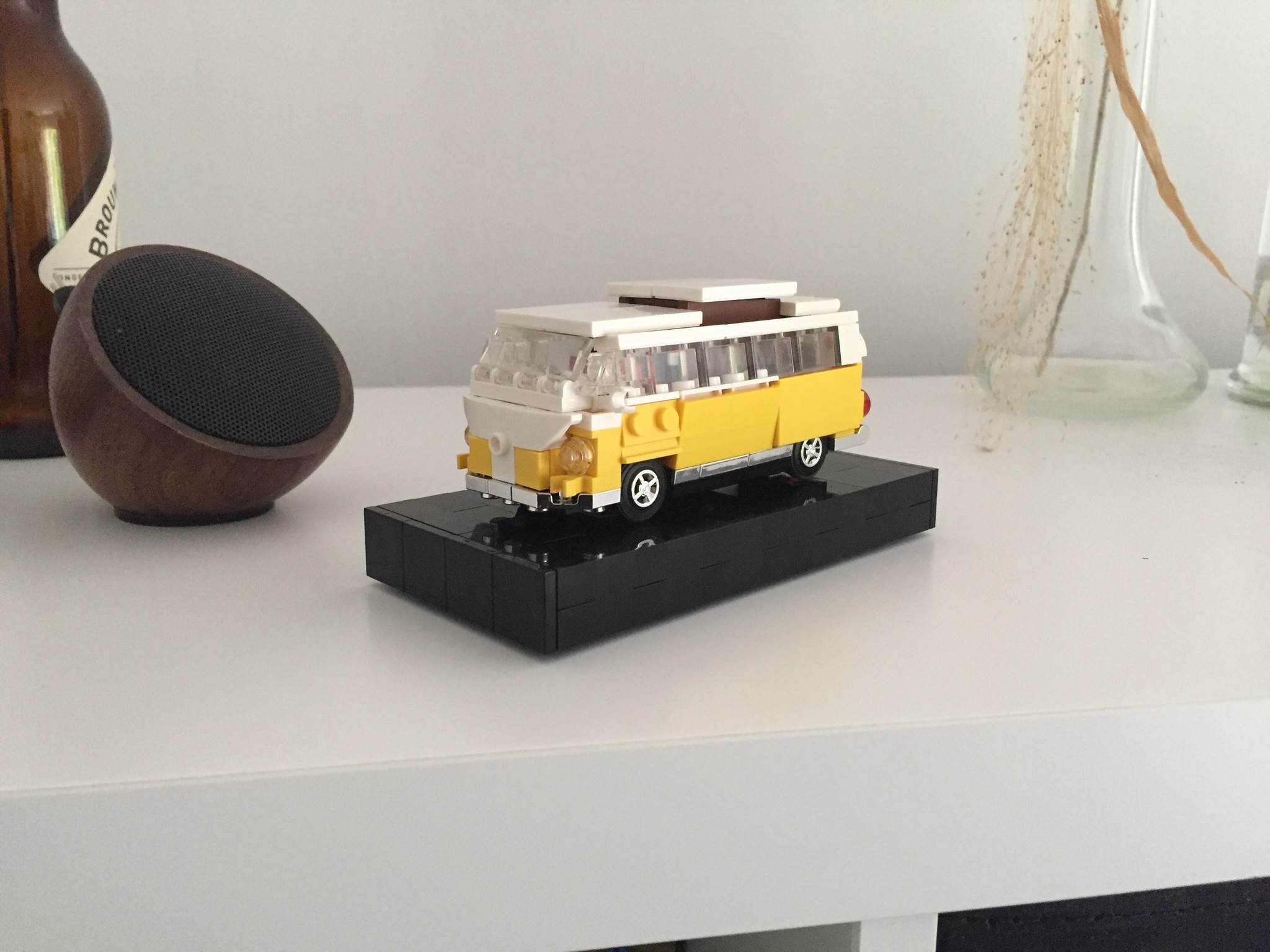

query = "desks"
[{"left": 0, "top": 367, "right": 1270, "bottom": 952}]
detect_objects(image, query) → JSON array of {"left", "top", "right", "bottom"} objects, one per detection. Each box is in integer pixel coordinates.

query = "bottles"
[
  {"left": 0, "top": 0, "right": 121, "bottom": 460},
  {"left": 1223, "top": 179, "right": 1270, "bottom": 408},
  {"left": 986, "top": 0, "right": 1212, "bottom": 416}
]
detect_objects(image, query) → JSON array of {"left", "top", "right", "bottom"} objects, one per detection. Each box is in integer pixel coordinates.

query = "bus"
[{"left": 456, "top": 279, "right": 871, "bottom": 522}]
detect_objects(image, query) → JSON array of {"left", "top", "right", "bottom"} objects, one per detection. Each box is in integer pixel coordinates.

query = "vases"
[{"left": 988, "top": 0, "right": 1208, "bottom": 413}]
